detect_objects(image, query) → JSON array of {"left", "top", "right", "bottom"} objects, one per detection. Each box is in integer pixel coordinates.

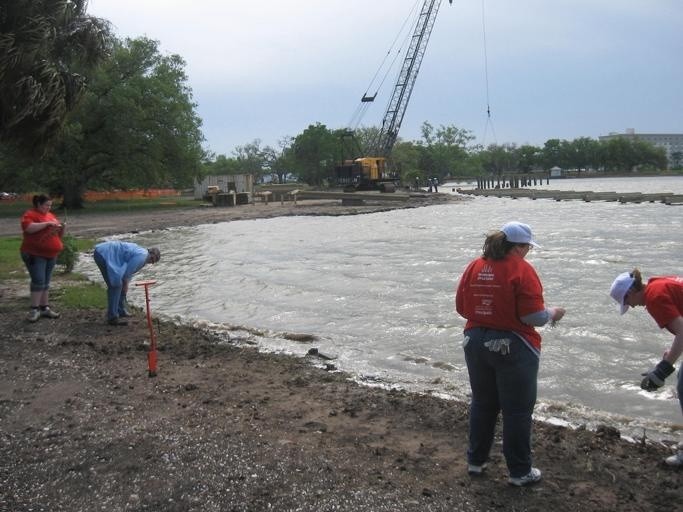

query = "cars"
[{"left": 0, "top": 191, "right": 15, "bottom": 201}]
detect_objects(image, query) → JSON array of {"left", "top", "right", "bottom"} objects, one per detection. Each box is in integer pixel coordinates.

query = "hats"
[
  {"left": 501, "top": 222, "right": 542, "bottom": 250},
  {"left": 609, "top": 273, "right": 638, "bottom": 316},
  {"left": 148, "top": 247, "right": 160, "bottom": 261}
]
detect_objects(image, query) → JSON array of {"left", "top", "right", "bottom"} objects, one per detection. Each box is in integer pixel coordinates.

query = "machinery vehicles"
[
  {"left": 202, "top": 185, "right": 225, "bottom": 203},
  {"left": 333, "top": 0, "right": 494, "bottom": 194}
]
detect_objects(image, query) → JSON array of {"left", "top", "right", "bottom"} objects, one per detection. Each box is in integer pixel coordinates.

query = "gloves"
[{"left": 640, "top": 361, "right": 676, "bottom": 392}]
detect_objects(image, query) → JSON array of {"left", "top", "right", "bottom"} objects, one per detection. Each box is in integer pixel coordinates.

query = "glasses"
[{"left": 151, "top": 254, "right": 154, "bottom": 265}]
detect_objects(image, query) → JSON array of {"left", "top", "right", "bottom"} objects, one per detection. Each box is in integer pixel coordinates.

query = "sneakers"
[
  {"left": 468, "top": 464, "right": 488, "bottom": 474},
  {"left": 26, "top": 310, "right": 40, "bottom": 322},
  {"left": 108, "top": 318, "right": 128, "bottom": 326},
  {"left": 508, "top": 467, "right": 543, "bottom": 486},
  {"left": 120, "top": 311, "right": 133, "bottom": 317},
  {"left": 40, "top": 308, "right": 61, "bottom": 318},
  {"left": 666, "top": 453, "right": 683, "bottom": 467}
]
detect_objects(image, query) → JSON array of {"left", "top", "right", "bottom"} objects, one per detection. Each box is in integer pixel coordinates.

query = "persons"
[
  {"left": 93, "top": 241, "right": 160, "bottom": 325},
  {"left": 609, "top": 269, "right": 683, "bottom": 466},
  {"left": 20, "top": 194, "right": 67, "bottom": 323},
  {"left": 414, "top": 177, "right": 438, "bottom": 193},
  {"left": 455, "top": 221, "right": 566, "bottom": 486}
]
